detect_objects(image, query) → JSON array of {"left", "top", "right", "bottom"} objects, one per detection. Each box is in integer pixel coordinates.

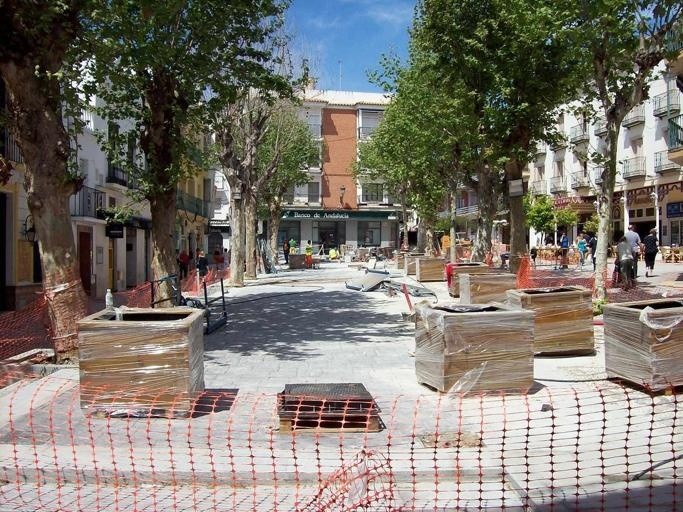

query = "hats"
[{"left": 198, "top": 251, "right": 206, "bottom": 258}]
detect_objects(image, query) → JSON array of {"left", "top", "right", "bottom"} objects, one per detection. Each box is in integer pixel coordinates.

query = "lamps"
[{"left": 19, "top": 214, "right": 37, "bottom": 243}]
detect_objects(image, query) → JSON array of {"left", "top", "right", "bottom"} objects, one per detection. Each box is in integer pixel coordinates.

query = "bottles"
[{"left": 104, "top": 288, "right": 113, "bottom": 312}]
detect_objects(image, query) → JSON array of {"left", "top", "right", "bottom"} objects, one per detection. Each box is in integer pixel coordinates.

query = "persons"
[
  {"left": 561, "top": 222, "right": 660, "bottom": 292},
  {"left": 175, "top": 237, "right": 341, "bottom": 279}
]
